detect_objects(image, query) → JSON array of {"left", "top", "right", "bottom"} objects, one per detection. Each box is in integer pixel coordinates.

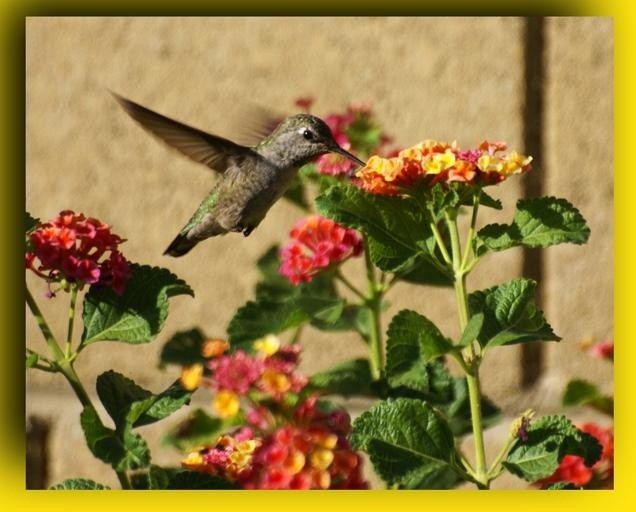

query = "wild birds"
[{"left": 101, "top": 86, "right": 367, "bottom": 259}]
